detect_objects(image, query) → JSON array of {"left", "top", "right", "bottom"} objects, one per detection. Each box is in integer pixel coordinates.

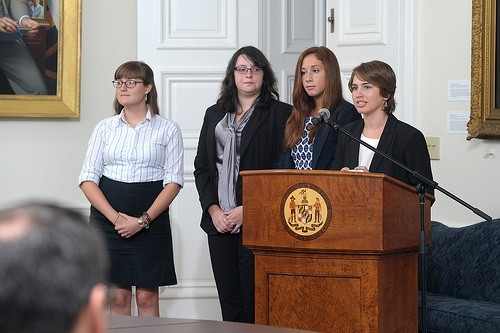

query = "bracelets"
[{"left": 138, "top": 211, "right": 153, "bottom": 229}]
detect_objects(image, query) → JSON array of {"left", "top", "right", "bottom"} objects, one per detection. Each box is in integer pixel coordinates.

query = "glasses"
[
  {"left": 234, "top": 66, "right": 265, "bottom": 73},
  {"left": 112, "top": 79, "right": 146, "bottom": 88}
]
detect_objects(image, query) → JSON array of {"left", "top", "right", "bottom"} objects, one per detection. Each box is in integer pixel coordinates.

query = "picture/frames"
[
  {"left": 466, "top": 0, "right": 500, "bottom": 140},
  {"left": 0, "top": 0, "right": 81, "bottom": 119}
]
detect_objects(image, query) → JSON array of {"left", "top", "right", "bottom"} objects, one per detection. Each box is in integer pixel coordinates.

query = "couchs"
[{"left": 415, "top": 218, "right": 500, "bottom": 333}]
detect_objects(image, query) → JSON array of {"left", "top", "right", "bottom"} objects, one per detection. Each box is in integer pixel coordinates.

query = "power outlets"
[{"left": 425, "top": 137, "right": 440, "bottom": 159}]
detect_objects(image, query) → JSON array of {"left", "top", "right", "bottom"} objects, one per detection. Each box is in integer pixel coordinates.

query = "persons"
[
  {"left": 77, "top": 61, "right": 185, "bottom": 317},
  {"left": 194, "top": 46, "right": 293, "bottom": 324},
  {"left": 0, "top": 198, "right": 114, "bottom": 333},
  {"left": 330, "top": 61, "right": 436, "bottom": 206},
  {"left": 0, "top": 0, "right": 50, "bottom": 96},
  {"left": 280, "top": 46, "right": 361, "bottom": 170}
]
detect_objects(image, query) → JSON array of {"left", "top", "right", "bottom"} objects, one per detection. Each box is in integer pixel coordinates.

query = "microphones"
[{"left": 312, "top": 108, "right": 330, "bottom": 125}]
